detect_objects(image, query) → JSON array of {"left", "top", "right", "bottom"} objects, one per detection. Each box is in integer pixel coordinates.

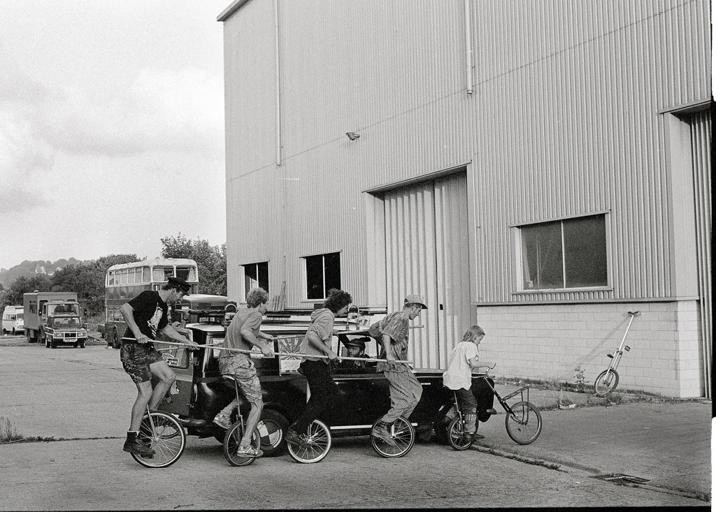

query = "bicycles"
[
  {"left": 369, "top": 415, "right": 415, "bottom": 457},
  {"left": 222, "top": 375, "right": 261, "bottom": 466},
  {"left": 470, "top": 367, "right": 543, "bottom": 445},
  {"left": 594, "top": 309, "right": 638, "bottom": 398},
  {"left": 130, "top": 403, "right": 186, "bottom": 468},
  {"left": 446, "top": 387, "right": 479, "bottom": 451},
  {"left": 287, "top": 367, "right": 332, "bottom": 464}
]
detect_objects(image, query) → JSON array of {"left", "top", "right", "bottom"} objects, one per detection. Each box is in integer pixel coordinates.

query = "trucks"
[{"left": 23, "top": 292, "right": 79, "bottom": 343}]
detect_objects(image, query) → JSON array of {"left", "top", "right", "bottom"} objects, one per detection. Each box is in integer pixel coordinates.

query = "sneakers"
[
  {"left": 212, "top": 412, "right": 232, "bottom": 430},
  {"left": 123, "top": 430, "right": 155, "bottom": 455},
  {"left": 284, "top": 429, "right": 310, "bottom": 450},
  {"left": 237, "top": 441, "right": 264, "bottom": 458},
  {"left": 370, "top": 422, "right": 395, "bottom": 447},
  {"left": 465, "top": 432, "right": 485, "bottom": 439}
]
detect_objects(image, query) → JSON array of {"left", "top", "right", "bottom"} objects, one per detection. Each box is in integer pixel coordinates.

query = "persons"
[
  {"left": 284, "top": 288, "right": 351, "bottom": 448},
  {"left": 119, "top": 278, "right": 191, "bottom": 455},
  {"left": 335, "top": 339, "right": 365, "bottom": 374},
  {"left": 371, "top": 295, "right": 428, "bottom": 448},
  {"left": 212, "top": 288, "right": 274, "bottom": 459},
  {"left": 444, "top": 325, "right": 496, "bottom": 441}
]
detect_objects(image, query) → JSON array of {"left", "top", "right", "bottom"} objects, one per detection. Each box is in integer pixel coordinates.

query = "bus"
[
  {"left": 104, "top": 259, "right": 199, "bottom": 349},
  {"left": 2, "top": 306, "right": 24, "bottom": 335}
]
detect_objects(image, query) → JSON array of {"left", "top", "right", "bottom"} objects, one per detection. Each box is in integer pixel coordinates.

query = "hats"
[
  {"left": 167, "top": 276, "right": 191, "bottom": 292},
  {"left": 348, "top": 337, "right": 365, "bottom": 349},
  {"left": 404, "top": 294, "right": 428, "bottom": 310}
]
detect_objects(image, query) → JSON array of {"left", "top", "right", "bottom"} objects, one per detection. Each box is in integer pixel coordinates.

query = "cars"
[{"left": 44, "top": 312, "right": 88, "bottom": 348}]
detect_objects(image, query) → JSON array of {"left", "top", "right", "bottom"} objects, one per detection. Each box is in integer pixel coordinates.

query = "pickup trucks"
[{"left": 177, "top": 315, "right": 496, "bottom": 456}]
